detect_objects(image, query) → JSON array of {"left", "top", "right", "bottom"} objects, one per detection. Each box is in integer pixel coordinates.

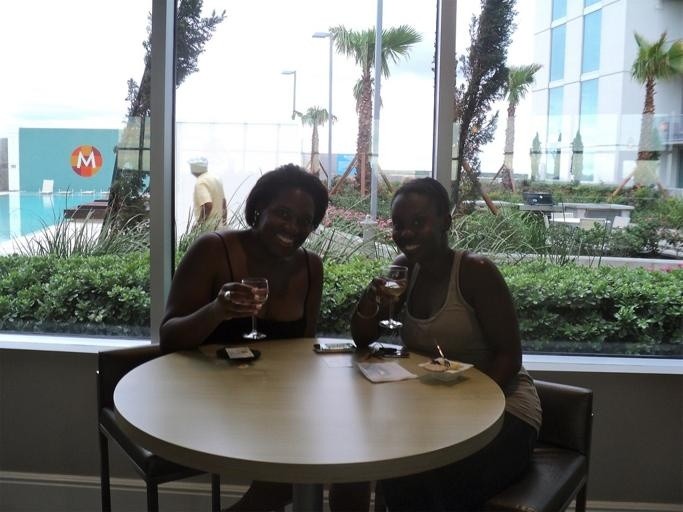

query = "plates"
[{"left": 419, "top": 357, "right": 475, "bottom": 381}]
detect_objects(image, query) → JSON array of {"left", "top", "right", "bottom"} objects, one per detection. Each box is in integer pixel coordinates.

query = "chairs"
[
  {"left": 94, "top": 348, "right": 221, "bottom": 511},
  {"left": 476, "top": 376, "right": 594, "bottom": 511},
  {"left": 42, "top": 197, "right": 54, "bottom": 207},
  {"left": 37, "top": 179, "right": 53, "bottom": 195},
  {"left": 544, "top": 211, "right": 636, "bottom": 248}
]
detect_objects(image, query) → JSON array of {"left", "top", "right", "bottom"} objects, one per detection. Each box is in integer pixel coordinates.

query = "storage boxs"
[{"left": 522, "top": 190, "right": 552, "bottom": 206}]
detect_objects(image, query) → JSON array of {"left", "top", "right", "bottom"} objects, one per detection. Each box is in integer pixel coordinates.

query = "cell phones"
[
  {"left": 217, "top": 346, "right": 260, "bottom": 361},
  {"left": 314, "top": 343, "right": 355, "bottom": 353}
]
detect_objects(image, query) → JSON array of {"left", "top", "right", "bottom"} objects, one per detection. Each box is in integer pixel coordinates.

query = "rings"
[{"left": 223, "top": 290, "right": 232, "bottom": 301}]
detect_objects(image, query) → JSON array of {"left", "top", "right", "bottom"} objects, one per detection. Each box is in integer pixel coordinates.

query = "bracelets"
[{"left": 356, "top": 303, "right": 379, "bottom": 320}]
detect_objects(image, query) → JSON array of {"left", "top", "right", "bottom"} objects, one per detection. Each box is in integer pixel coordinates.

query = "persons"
[
  {"left": 157, "top": 162, "right": 371, "bottom": 512},
  {"left": 347, "top": 175, "right": 544, "bottom": 511},
  {"left": 184, "top": 153, "right": 228, "bottom": 233}
]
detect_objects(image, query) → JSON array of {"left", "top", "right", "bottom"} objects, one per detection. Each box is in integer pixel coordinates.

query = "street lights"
[
  {"left": 280, "top": 69, "right": 296, "bottom": 119},
  {"left": 311, "top": 32, "right": 333, "bottom": 197}
]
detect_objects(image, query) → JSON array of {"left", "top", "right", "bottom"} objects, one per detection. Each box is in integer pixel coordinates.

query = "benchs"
[{"left": 60, "top": 188, "right": 110, "bottom": 219}]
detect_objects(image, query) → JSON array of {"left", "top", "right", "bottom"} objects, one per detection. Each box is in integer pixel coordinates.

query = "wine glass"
[
  {"left": 377, "top": 264, "right": 408, "bottom": 329},
  {"left": 238, "top": 275, "right": 269, "bottom": 341}
]
